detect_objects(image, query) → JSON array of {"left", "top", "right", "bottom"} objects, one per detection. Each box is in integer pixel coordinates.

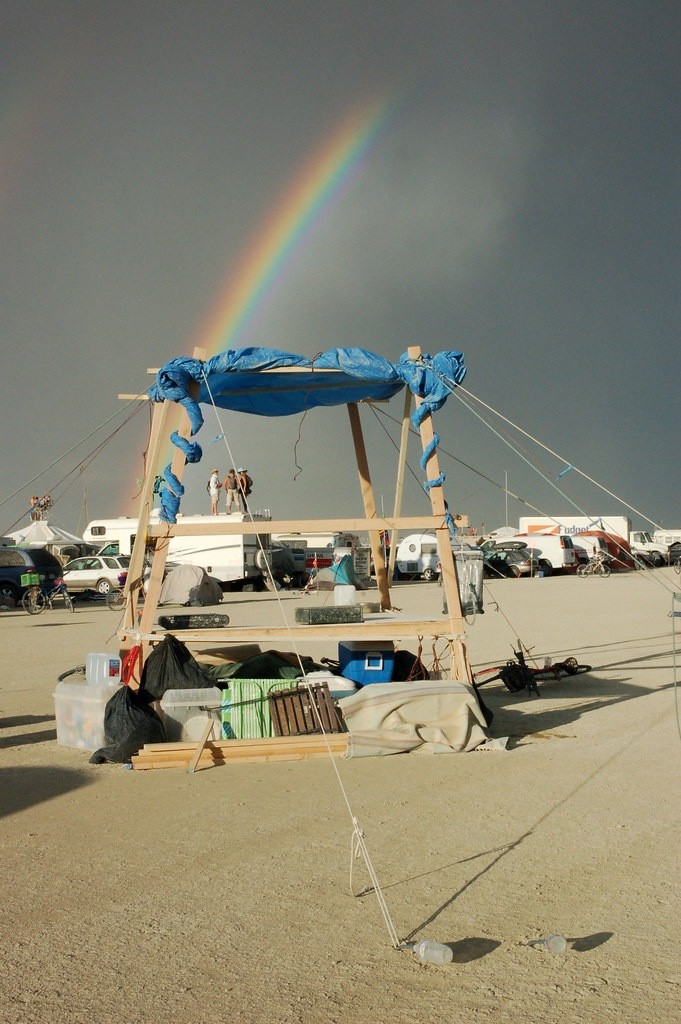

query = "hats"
[
  {"left": 236, "top": 468, "right": 246, "bottom": 473},
  {"left": 211, "top": 469, "right": 217, "bottom": 474}
]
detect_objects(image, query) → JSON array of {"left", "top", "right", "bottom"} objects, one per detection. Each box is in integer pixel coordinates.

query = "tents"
[
  {"left": 570, "top": 530, "right": 636, "bottom": 572},
  {"left": 309, "top": 555, "right": 369, "bottom": 589},
  {"left": 159, "top": 565, "right": 223, "bottom": 608},
  {"left": 2, "top": 519, "right": 85, "bottom": 558}
]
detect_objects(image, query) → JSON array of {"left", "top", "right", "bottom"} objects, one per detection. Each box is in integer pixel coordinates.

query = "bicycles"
[
  {"left": 574, "top": 554, "right": 611, "bottom": 579},
  {"left": 106, "top": 588, "right": 127, "bottom": 611},
  {"left": 472, "top": 638, "right": 593, "bottom": 697},
  {"left": 631, "top": 547, "right": 645, "bottom": 571},
  {"left": 21, "top": 570, "right": 74, "bottom": 615},
  {"left": 674, "top": 555, "right": 681, "bottom": 574}
]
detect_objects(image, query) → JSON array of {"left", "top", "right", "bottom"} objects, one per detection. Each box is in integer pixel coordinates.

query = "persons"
[
  {"left": 207, "top": 469, "right": 223, "bottom": 517},
  {"left": 238, "top": 466, "right": 253, "bottom": 514},
  {"left": 30, "top": 495, "right": 54, "bottom": 509},
  {"left": 225, "top": 468, "right": 241, "bottom": 515}
]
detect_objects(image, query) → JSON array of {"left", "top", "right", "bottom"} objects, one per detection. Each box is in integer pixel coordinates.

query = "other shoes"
[
  {"left": 210, "top": 512, "right": 218, "bottom": 516},
  {"left": 225, "top": 513, "right": 230, "bottom": 515}
]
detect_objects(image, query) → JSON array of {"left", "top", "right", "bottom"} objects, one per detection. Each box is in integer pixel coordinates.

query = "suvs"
[{"left": 29, "top": 540, "right": 88, "bottom": 558}]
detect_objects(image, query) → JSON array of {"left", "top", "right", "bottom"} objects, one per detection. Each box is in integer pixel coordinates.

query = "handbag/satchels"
[{"left": 206, "top": 481, "right": 210, "bottom": 496}]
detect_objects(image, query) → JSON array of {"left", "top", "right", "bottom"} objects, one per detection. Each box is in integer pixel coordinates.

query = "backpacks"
[
  {"left": 242, "top": 475, "right": 252, "bottom": 487},
  {"left": 227, "top": 475, "right": 236, "bottom": 489}
]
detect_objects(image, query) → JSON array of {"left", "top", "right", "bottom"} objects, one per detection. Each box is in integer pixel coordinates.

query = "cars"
[{"left": 485, "top": 551, "right": 540, "bottom": 578}]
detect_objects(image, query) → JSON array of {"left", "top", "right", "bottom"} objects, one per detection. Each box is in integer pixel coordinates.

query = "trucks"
[
  {"left": 569, "top": 535, "right": 608, "bottom": 563},
  {"left": 291, "top": 547, "right": 372, "bottom": 585},
  {"left": 82, "top": 513, "right": 272, "bottom": 585},
  {"left": 481, "top": 534, "right": 577, "bottom": 576},
  {"left": 396, "top": 533, "right": 474, "bottom": 581},
  {"left": 518, "top": 516, "right": 670, "bottom": 569},
  {"left": 654, "top": 529, "right": 681, "bottom": 546},
  {"left": 271, "top": 533, "right": 360, "bottom": 548}
]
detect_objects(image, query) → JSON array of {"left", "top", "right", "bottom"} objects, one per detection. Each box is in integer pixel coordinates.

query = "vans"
[
  {"left": 0, "top": 547, "right": 61, "bottom": 608},
  {"left": 60, "top": 555, "right": 132, "bottom": 595}
]
